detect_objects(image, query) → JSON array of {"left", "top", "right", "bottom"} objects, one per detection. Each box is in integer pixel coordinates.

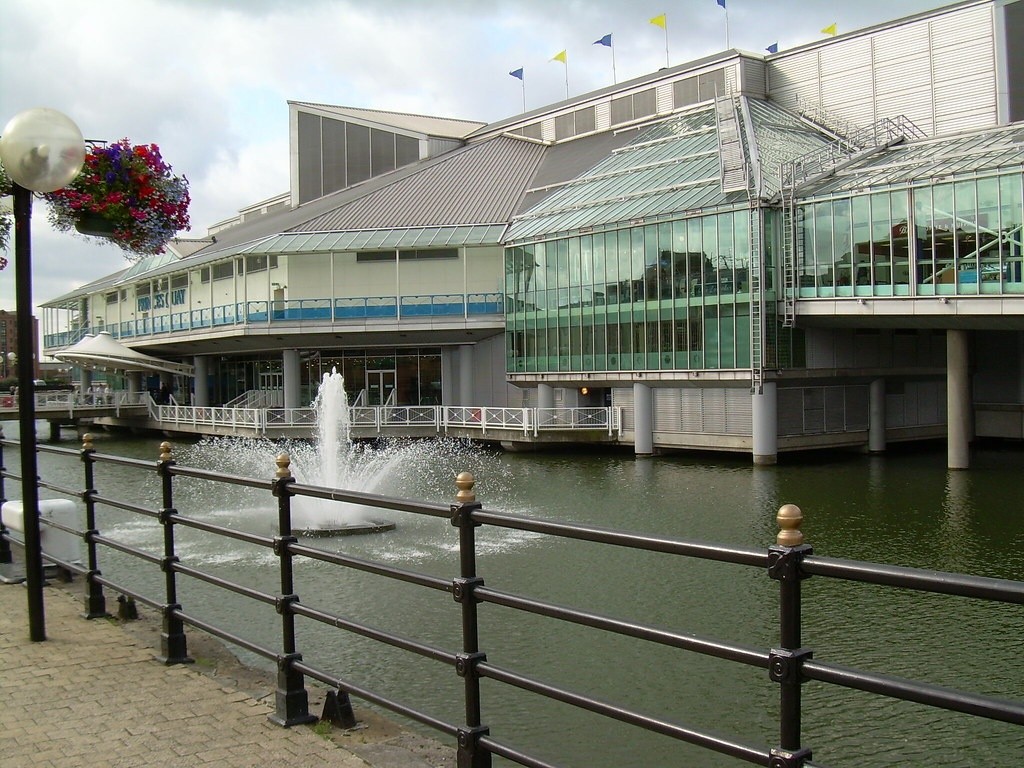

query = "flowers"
[
  {"left": 44, "top": 138, "right": 192, "bottom": 263},
  {"left": 0, "top": 162, "right": 15, "bottom": 272}
]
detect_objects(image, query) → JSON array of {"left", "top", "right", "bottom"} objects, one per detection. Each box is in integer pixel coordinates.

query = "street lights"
[
  {"left": 0, "top": 104, "right": 87, "bottom": 642},
  {"left": 1, "top": 352, "right": 17, "bottom": 379}
]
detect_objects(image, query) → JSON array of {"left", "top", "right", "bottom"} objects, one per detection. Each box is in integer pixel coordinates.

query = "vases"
[{"left": 72, "top": 213, "right": 116, "bottom": 238}]
